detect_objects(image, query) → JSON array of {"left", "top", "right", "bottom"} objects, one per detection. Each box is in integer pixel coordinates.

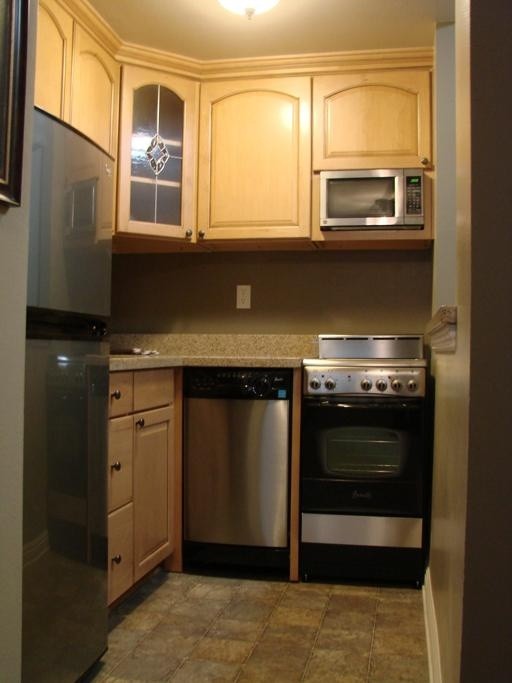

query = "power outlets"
[{"left": 235, "top": 283, "right": 252, "bottom": 312}]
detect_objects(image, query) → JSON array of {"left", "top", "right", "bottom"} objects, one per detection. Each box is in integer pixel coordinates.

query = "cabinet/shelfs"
[
  {"left": 198, "top": 76, "right": 312, "bottom": 240},
  {"left": 34, "top": 0, "right": 118, "bottom": 162},
  {"left": 105, "top": 369, "right": 175, "bottom": 615},
  {"left": 118, "top": 63, "right": 198, "bottom": 240},
  {"left": 312, "top": 71, "right": 435, "bottom": 169}
]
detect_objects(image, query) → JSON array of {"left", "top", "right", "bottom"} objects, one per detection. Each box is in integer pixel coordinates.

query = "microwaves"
[{"left": 318, "top": 166, "right": 426, "bottom": 231}]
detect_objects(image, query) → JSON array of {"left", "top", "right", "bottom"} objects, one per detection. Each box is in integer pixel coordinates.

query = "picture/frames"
[{"left": 1, "top": 0, "right": 29, "bottom": 203}]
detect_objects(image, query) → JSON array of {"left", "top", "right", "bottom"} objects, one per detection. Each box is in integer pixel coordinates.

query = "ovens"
[{"left": 301, "top": 367, "right": 429, "bottom": 551}]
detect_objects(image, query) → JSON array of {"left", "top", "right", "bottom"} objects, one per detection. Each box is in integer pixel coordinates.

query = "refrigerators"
[{"left": 1, "top": 105, "right": 116, "bottom": 683}]
